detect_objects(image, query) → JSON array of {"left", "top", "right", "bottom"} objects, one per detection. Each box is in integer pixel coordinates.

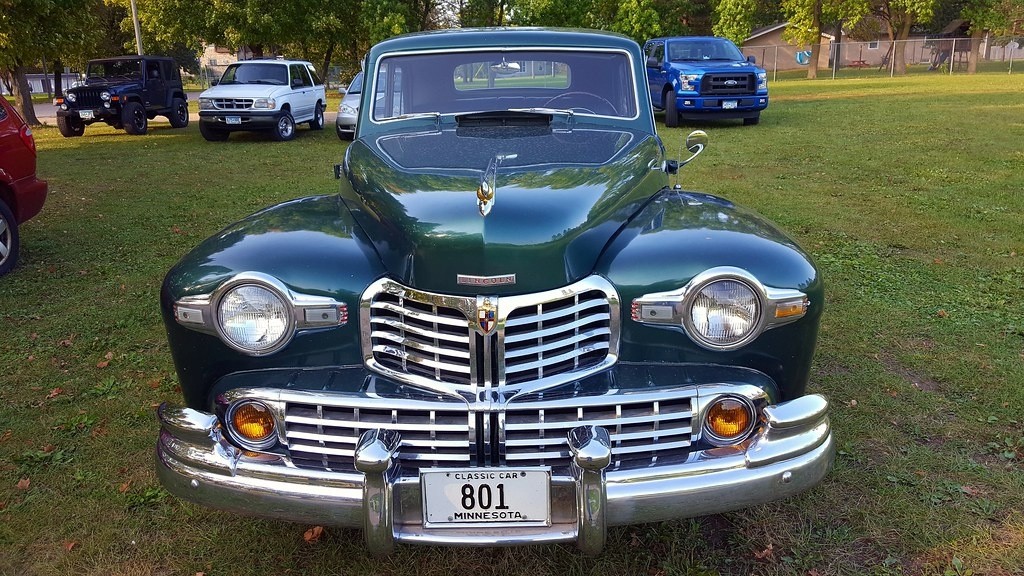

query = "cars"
[
  {"left": 0, "top": 93, "right": 49, "bottom": 277},
  {"left": 336, "top": 68, "right": 403, "bottom": 140},
  {"left": 156, "top": 23, "right": 834, "bottom": 558}
]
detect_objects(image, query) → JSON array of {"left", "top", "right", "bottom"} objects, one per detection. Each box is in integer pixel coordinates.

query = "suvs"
[
  {"left": 54, "top": 56, "right": 190, "bottom": 137},
  {"left": 644, "top": 36, "right": 769, "bottom": 128},
  {"left": 199, "top": 58, "right": 327, "bottom": 143}
]
